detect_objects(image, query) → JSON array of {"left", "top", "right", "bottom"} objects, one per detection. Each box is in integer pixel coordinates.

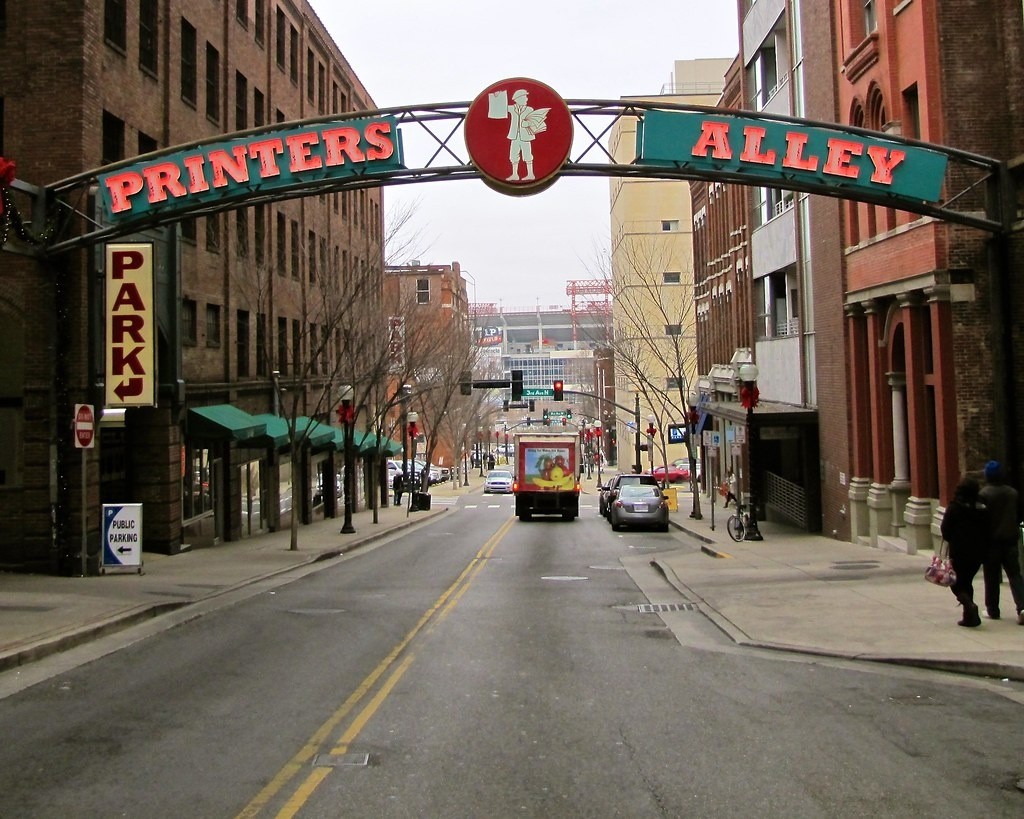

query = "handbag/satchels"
[{"left": 924, "top": 555, "right": 957, "bottom": 588}]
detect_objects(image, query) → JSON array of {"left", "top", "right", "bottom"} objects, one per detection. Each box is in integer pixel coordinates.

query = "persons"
[
  {"left": 470, "top": 453, "right": 495, "bottom": 468},
  {"left": 941, "top": 460, "right": 1024, "bottom": 626},
  {"left": 589, "top": 452, "right": 604, "bottom": 472},
  {"left": 724, "top": 470, "right": 738, "bottom": 507},
  {"left": 392, "top": 469, "right": 403, "bottom": 505}
]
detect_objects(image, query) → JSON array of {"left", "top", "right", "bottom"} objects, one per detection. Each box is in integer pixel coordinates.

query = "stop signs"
[{"left": 76, "top": 405, "right": 94, "bottom": 446}]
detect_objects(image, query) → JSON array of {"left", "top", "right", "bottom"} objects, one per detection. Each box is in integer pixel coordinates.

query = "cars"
[
  {"left": 597, "top": 456, "right": 701, "bottom": 533},
  {"left": 315, "top": 473, "right": 341, "bottom": 497},
  {"left": 483, "top": 470, "right": 515, "bottom": 493}
]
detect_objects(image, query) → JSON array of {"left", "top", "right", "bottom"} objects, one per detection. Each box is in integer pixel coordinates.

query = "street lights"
[
  {"left": 408, "top": 411, "right": 421, "bottom": 512},
  {"left": 585, "top": 419, "right": 603, "bottom": 488},
  {"left": 462, "top": 423, "right": 470, "bottom": 486},
  {"left": 739, "top": 362, "right": 765, "bottom": 542},
  {"left": 648, "top": 413, "right": 654, "bottom": 474},
  {"left": 476, "top": 422, "right": 511, "bottom": 477},
  {"left": 338, "top": 384, "right": 361, "bottom": 535},
  {"left": 686, "top": 391, "right": 705, "bottom": 519}
]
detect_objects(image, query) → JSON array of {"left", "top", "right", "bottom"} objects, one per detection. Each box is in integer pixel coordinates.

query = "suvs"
[{"left": 342, "top": 458, "right": 450, "bottom": 489}]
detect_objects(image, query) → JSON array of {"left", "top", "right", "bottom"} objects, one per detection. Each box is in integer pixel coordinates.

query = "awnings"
[{"left": 189, "top": 404, "right": 402, "bottom": 458}]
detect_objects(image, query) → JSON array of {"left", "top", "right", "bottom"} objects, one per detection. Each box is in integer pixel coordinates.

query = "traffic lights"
[
  {"left": 562, "top": 418, "right": 566, "bottom": 426},
  {"left": 567, "top": 409, "right": 572, "bottom": 419},
  {"left": 546, "top": 418, "right": 550, "bottom": 426},
  {"left": 553, "top": 380, "right": 563, "bottom": 401},
  {"left": 543, "top": 409, "right": 548, "bottom": 425}
]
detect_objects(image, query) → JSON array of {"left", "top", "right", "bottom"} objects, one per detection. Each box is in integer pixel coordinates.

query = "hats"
[{"left": 984, "top": 461, "right": 1001, "bottom": 479}]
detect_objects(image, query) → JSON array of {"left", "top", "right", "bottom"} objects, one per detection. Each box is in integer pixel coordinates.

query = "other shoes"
[
  {"left": 982, "top": 610, "right": 992, "bottom": 620},
  {"left": 1016, "top": 610, "right": 1024, "bottom": 625}
]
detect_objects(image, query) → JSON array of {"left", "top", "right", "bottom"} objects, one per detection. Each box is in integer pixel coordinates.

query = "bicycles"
[{"left": 726, "top": 503, "right": 749, "bottom": 542}]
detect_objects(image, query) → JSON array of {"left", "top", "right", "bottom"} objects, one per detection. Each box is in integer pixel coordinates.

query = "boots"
[{"left": 958, "top": 604, "right": 981, "bottom": 628}]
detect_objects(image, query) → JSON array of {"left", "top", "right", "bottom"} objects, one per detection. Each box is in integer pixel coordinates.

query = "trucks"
[{"left": 513, "top": 433, "right": 584, "bottom": 520}]
